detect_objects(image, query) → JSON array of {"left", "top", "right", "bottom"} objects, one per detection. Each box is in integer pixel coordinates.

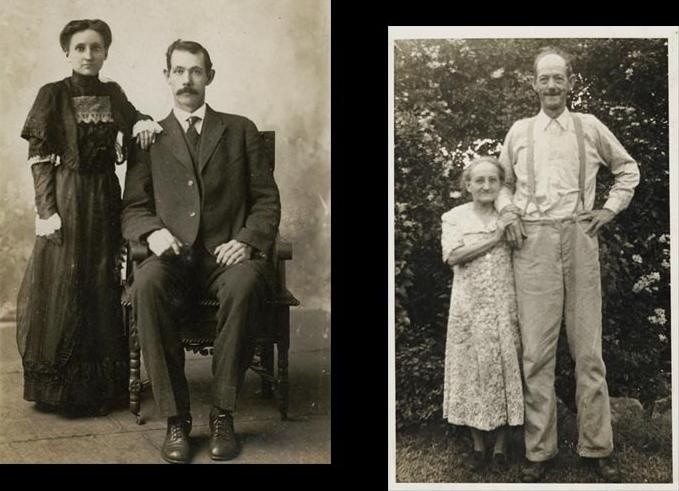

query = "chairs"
[{"left": 120, "top": 131, "right": 300, "bottom": 419}]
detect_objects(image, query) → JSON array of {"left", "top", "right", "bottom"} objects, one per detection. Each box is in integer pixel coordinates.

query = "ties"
[{"left": 185, "top": 117, "right": 199, "bottom": 151}]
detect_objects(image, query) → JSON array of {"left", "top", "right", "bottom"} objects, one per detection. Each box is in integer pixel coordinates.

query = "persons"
[
  {"left": 13, "top": 17, "right": 163, "bottom": 422},
  {"left": 118, "top": 38, "right": 282, "bottom": 464},
  {"left": 442, "top": 156, "right": 526, "bottom": 472},
  {"left": 493, "top": 47, "right": 642, "bottom": 483}
]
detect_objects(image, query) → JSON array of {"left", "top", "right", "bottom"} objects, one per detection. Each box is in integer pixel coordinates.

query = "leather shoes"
[
  {"left": 209, "top": 410, "right": 239, "bottom": 460},
  {"left": 467, "top": 450, "right": 506, "bottom": 469},
  {"left": 521, "top": 461, "right": 549, "bottom": 482},
  {"left": 161, "top": 415, "right": 192, "bottom": 464},
  {"left": 584, "top": 459, "right": 619, "bottom": 481}
]
